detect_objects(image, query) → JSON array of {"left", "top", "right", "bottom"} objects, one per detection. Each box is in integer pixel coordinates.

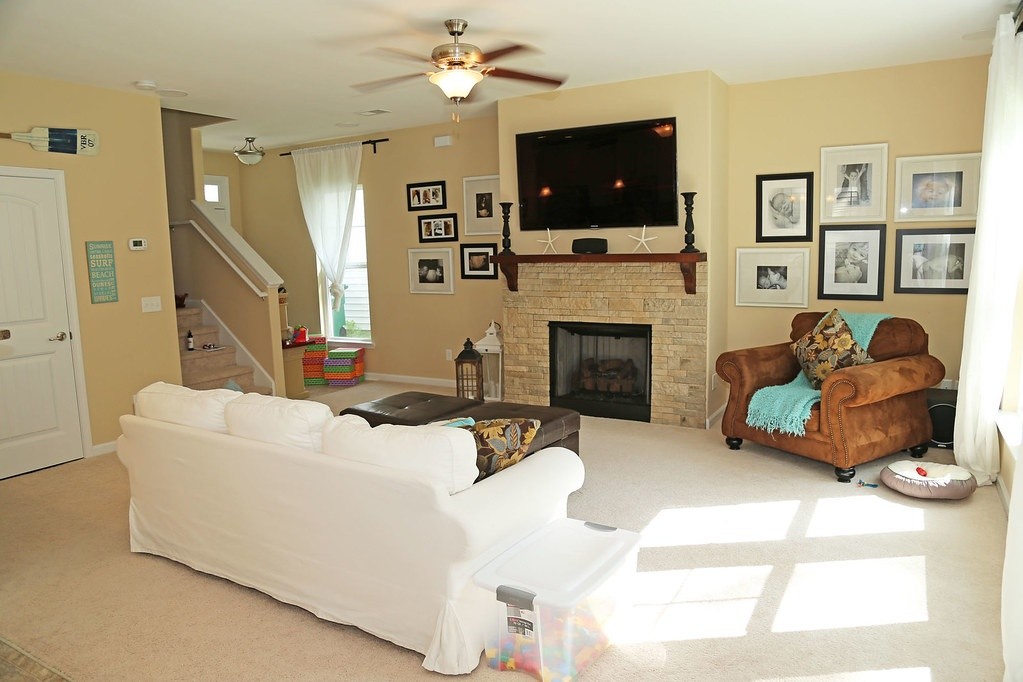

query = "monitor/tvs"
[{"left": 516, "top": 116, "right": 678, "bottom": 232}]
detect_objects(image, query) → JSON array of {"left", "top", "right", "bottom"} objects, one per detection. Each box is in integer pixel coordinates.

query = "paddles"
[{"left": 0, "top": 127, "right": 100, "bottom": 157}]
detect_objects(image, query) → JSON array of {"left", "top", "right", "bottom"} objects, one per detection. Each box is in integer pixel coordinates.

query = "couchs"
[
  {"left": 716, "top": 312, "right": 945, "bottom": 483},
  {"left": 114, "top": 382, "right": 584, "bottom": 676}
]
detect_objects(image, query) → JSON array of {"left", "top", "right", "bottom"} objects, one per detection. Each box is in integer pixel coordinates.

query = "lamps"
[{"left": 454, "top": 320, "right": 505, "bottom": 402}]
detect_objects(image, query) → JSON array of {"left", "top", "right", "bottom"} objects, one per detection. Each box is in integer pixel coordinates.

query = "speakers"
[{"left": 572, "top": 238, "right": 608, "bottom": 254}]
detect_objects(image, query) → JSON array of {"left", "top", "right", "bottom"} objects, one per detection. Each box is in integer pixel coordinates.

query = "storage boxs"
[{"left": 472, "top": 517, "right": 640, "bottom": 682}]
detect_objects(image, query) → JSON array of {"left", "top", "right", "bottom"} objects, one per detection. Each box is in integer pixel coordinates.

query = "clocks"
[
  {"left": 233, "top": 137, "right": 265, "bottom": 166},
  {"left": 429, "top": 61, "right": 483, "bottom": 122}
]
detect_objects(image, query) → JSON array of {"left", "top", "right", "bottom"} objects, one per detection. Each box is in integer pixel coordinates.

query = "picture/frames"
[
  {"left": 407, "top": 181, "right": 447, "bottom": 212},
  {"left": 460, "top": 243, "right": 497, "bottom": 280},
  {"left": 418, "top": 213, "right": 458, "bottom": 243},
  {"left": 463, "top": 175, "right": 501, "bottom": 235},
  {"left": 756, "top": 171, "right": 813, "bottom": 242},
  {"left": 894, "top": 152, "right": 981, "bottom": 222},
  {"left": 736, "top": 248, "right": 810, "bottom": 307},
  {"left": 820, "top": 143, "right": 888, "bottom": 222},
  {"left": 817, "top": 225, "right": 886, "bottom": 301},
  {"left": 894, "top": 228, "right": 976, "bottom": 295},
  {"left": 408, "top": 248, "right": 454, "bottom": 294}
]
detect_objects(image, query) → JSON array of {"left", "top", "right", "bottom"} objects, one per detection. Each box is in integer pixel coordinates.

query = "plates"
[{"left": 194, "top": 346, "right": 225, "bottom": 352}]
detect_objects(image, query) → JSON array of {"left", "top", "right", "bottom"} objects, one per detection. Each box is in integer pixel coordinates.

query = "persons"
[
  {"left": 476, "top": 195, "right": 489, "bottom": 217},
  {"left": 844, "top": 168, "right": 866, "bottom": 201},
  {"left": 412, "top": 189, "right": 441, "bottom": 205},
  {"left": 924, "top": 180, "right": 950, "bottom": 203},
  {"left": 757, "top": 266, "right": 787, "bottom": 289},
  {"left": 418, "top": 262, "right": 444, "bottom": 283},
  {"left": 834, "top": 242, "right": 869, "bottom": 283},
  {"left": 922, "top": 254, "right": 963, "bottom": 273},
  {"left": 771, "top": 193, "right": 801, "bottom": 223}
]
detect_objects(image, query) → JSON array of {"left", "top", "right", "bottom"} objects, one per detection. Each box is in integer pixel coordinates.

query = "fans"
[{"left": 351, "top": 19, "right": 562, "bottom": 92}]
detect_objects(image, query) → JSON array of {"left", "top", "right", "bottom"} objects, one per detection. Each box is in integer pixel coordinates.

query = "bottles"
[{"left": 187, "top": 331, "right": 194, "bottom": 351}]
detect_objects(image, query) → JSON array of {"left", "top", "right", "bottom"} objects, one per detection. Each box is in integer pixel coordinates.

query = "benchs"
[{"left": 340, "top": 391, "right": 581, "bottom": 484}]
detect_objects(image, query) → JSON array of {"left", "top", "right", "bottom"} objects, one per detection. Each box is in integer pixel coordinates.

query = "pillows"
[{"left": 789, "top": 307, "right": 875, "bottom": 390}]
